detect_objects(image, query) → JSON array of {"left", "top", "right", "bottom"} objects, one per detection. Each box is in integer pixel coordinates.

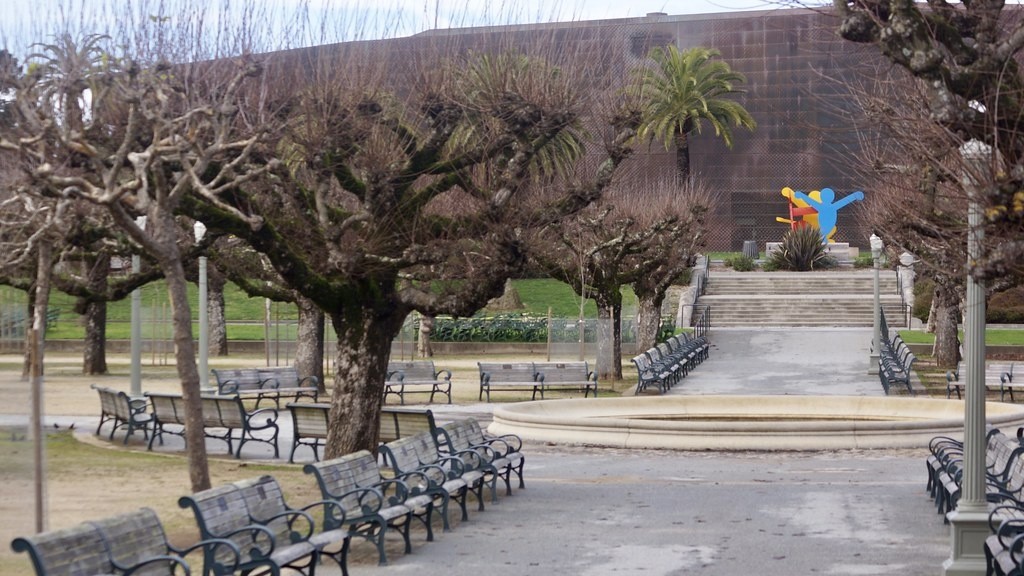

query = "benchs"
[
  {"left": 10, "top": 506, "right": 240, "bottom": 576},
  {"left": 946, "top": 360, "right": 1024, "bottom": 402},
  {"left": 285, "top": 403, "right": 447, "bottom": 464},
  {"left": 377, "top": 432, "right": 485, "bottom": 532},
  {"left": 178, "top": 475, "right": 355, "bottom": 576},
  {"left": 926, "top": 424, "right": 1024, "bottom": 576},
  {"left": 383, "top": 360, "right": 452, "bottom": 404},
  {"left": 432, "top": 418, "right": 525, "bottom": 505},
  {"left": 302, "top": 450, "right": 433, "bottom": 566},
  {"left": 143, "top": 392, "right": 281, "bottom": 459},
  {"left": 90, "top": 383, "right": 164, "bottom": 446},
  {"left": 631, "top": 332, "right": 711, "bottom": 395},
  {"left": 211, "top": 366, "right": 319, "bottom": 409},
  {"left": 477, "top": 361, "right": 598, "bottom": 403},
  {"left": 878, "top": 330, "right": 917, "bottom": 396}
]
